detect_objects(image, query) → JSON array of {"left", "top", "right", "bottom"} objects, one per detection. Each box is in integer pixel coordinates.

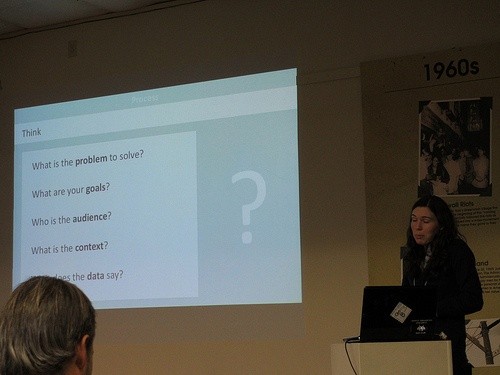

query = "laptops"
[{"left": 342, "top": 285, "right": 438, "bottom": 343}]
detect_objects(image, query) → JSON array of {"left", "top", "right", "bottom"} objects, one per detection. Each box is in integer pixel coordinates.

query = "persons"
[
  {"left": 0, "top": 276, "right": 96, "bottom": 375},
  {"left": 401, "top": 196, "right": 484, "bottom": 375},
  {"left": 420, "top": 138, "right": 490, "bottom": 196}
]
